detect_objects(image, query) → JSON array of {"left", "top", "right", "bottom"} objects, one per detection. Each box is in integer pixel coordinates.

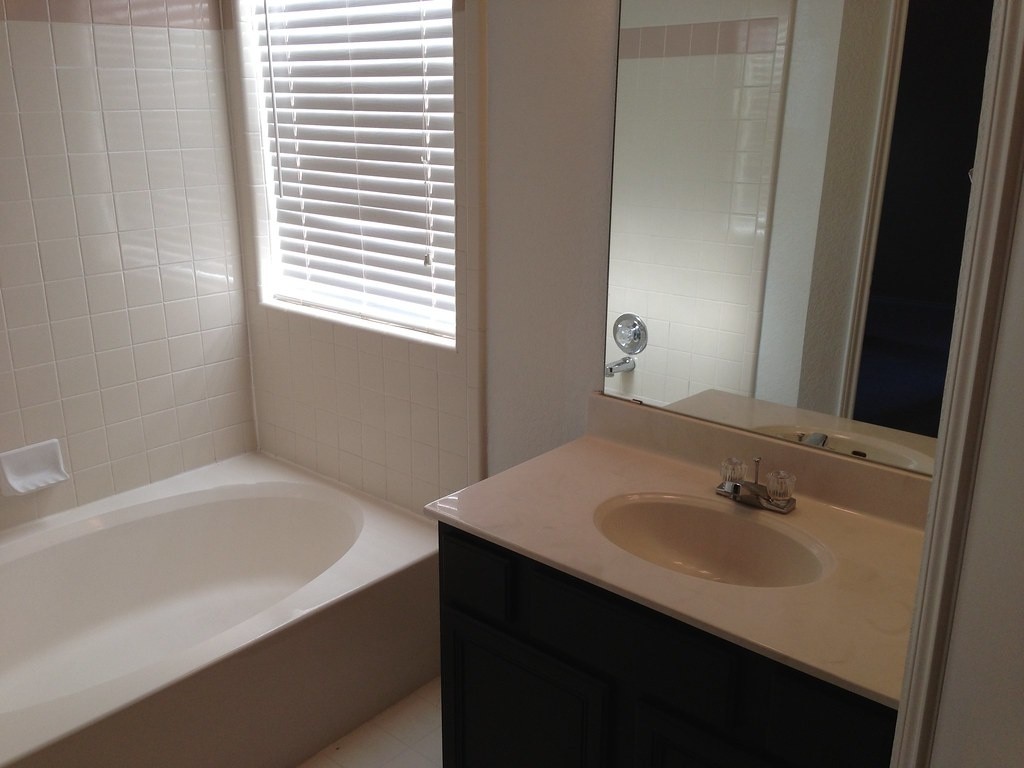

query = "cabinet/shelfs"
[{"left": 438, "top": 521, "right": 900, "bottom": 768}]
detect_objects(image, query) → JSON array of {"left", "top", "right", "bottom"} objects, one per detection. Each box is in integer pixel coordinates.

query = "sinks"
[
  {"left": 592, "top": 491, "right": 838, "bottom": 588},
  {"left": 751, "top": 425, "right": 934, "bottom": 479}
]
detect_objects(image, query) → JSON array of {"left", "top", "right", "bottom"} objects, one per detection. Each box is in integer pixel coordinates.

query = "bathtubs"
[{"left": 0, "top": 451, "right": 439, "bottom": 767}]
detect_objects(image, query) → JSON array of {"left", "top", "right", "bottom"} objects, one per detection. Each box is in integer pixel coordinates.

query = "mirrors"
[{"left": 604, "top": 0, "right": 993, "bottom": 477}]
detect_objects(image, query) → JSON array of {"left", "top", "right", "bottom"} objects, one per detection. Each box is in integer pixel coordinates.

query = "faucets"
[
  {"left": 714, "top": 456, "right": 797, "bottom": 515},
  {"left": 604, "top": 356, "right": 635, "bottom": 378},
  {"left": 793, "top": 432, "right": 829, "bottom": 447}
]
